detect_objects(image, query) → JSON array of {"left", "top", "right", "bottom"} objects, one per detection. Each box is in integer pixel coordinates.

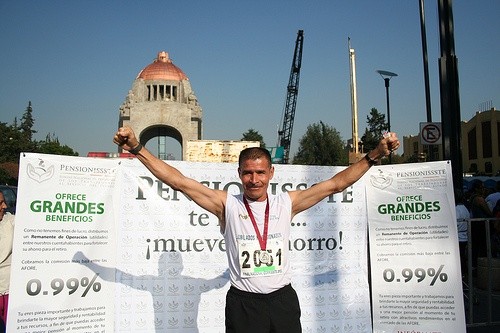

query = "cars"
[{"left": 0, "top": 185, "right": 17, "bottom": 211}]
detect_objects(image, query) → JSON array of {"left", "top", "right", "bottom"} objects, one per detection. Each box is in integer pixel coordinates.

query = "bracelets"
[
  {"left": 366, "top": 152, "right": 378, "bottom": 165},
  {"left": 129, "top": 144, "right": 143, "bottom": 154}
]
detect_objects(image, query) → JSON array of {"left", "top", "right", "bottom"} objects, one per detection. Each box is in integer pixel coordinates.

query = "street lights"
[{"left": 377, "top": 69, "right": 401, "bottom": 164}]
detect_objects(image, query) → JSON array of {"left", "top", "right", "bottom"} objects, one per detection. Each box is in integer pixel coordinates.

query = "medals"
[{"left": 259, "top": 251, "right": 270, "bottom": 263}]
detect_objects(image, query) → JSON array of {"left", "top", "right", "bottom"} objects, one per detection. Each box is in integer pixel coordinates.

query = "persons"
[
  {"left": 113, "top": 125, "right": 400, "bottom": 333},
  {"left": 0, "top": 192, "right": 15, "bottom": 324},
  {"left": 455, "top": 188, "right": 470, "bottom": 280},
  {"left": 466, "top": 179, "right": 500, "bottom": 258}
]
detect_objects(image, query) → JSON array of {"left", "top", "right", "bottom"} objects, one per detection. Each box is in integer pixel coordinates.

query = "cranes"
[{"left": 276, "top": 30, "right": 304, "bottom": 167}]
